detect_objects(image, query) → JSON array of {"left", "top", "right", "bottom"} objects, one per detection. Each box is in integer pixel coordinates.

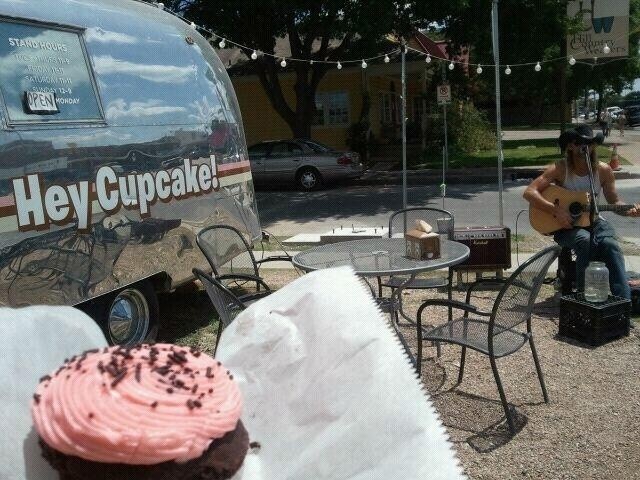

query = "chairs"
[
  {"left": 411, "top": 244, "right": 561, "bottom": 433},
  {"left": 193, "top": 268, "right": 278, "bottom": 364},
  {"left": 194, "top": 223, "right": 301, "bottom": 292},
  {"left": 387, "top": 206, "right": 454, "bottom": 325}
]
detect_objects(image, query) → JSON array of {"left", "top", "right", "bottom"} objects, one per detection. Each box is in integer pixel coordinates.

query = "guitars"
[{"left": 529, "top": 183, "right": 640, "bottom": 236}]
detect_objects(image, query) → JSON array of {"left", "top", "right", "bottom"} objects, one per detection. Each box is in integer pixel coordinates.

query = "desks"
[{"left": 292, "top": 235, "right": 471, "bottom": 371}]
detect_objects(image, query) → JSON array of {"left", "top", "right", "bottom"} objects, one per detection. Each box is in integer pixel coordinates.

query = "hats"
[{"left": 558, "top": 126, "right": 605, "bottom": 150}]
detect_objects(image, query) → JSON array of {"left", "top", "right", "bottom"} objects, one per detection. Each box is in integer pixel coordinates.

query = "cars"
[
  {"left": 577, "top": 102, "right": 640, "bottom": 127},
  {"left": 245, "top": 138, "right": 366, "bottom": 191}
]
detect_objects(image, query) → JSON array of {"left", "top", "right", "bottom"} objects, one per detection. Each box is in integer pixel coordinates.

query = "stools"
[{"left": 558, "top": 248, "right": 577, "bottom": 290}]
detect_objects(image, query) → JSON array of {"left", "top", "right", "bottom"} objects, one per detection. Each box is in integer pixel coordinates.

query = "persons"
[
  {"left": 599, "top": 108, "right": 628, "bottom": 137},
  {"left": 522, "top": 124, "right": 639, "bottom": 328}
]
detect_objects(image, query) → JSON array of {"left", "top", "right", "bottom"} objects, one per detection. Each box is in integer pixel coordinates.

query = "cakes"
[{"left": 30, "top": 342, "right": 248, "bottom": 480}]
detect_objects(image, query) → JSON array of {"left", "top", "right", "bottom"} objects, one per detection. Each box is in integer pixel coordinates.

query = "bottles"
[{"left": 583, "top": 261, "right": 610, "bottom": 302}]
